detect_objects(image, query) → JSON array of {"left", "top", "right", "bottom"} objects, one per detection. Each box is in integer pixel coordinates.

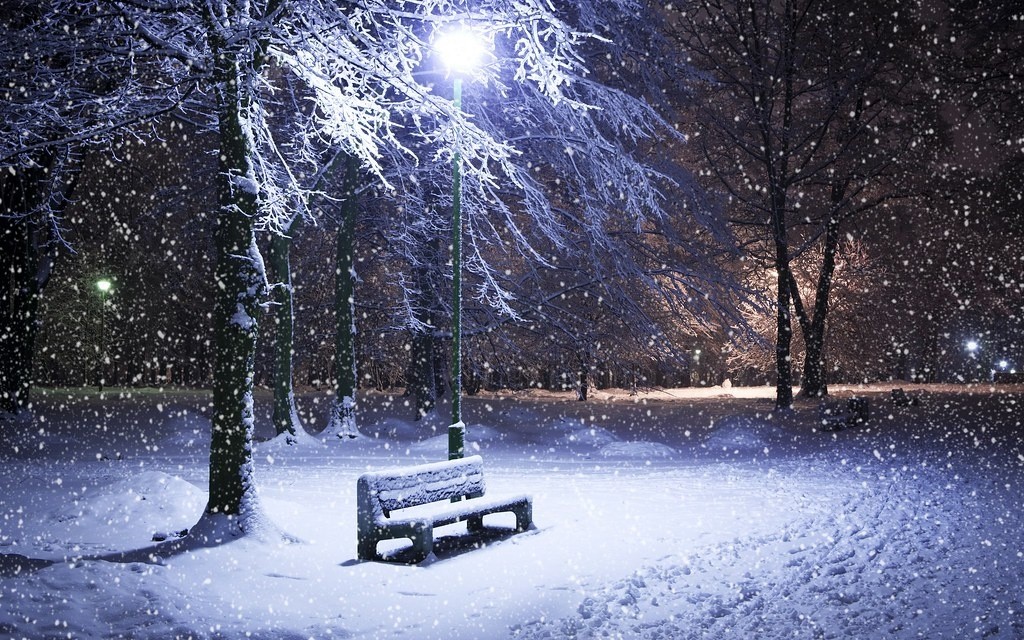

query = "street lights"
[
  {"left": 431, "top": 28, "right": 482, "bottom": 463},
  {"left": 97, "top": 280, "right": 111, "bottom": 391}
]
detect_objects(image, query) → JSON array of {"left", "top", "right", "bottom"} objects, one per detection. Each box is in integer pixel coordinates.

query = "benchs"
[
  {"left": 356, "top": 455, "right": 537, "bottom": 567},
  {"left": 995, "top": 372, "right": 1016, "bottom": 384},
  {"left": 818, "top": 402, "right": 857, "bottom": 432},
  {"left": 892, "top": 388, "right": 918, "bottom": 406}
]
